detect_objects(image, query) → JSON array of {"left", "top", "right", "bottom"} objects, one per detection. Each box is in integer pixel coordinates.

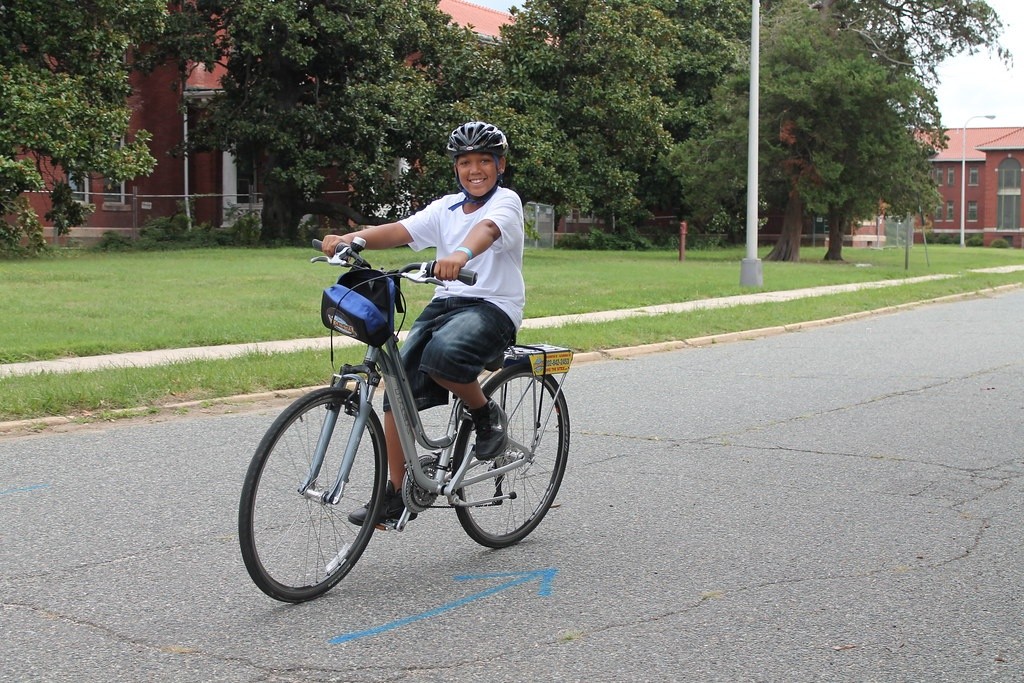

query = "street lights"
[{"left": 959, "top": 114, "right": 997, "bottom": 248}]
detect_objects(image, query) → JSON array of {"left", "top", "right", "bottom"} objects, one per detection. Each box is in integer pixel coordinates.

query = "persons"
[{"left": 320, "top": 120, "right": 526, "bottom": 529}]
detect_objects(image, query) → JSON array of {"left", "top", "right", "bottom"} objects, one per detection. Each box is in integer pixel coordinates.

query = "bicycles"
[{"left": 237, "top": 236, "right": 576, "bottom": 605}]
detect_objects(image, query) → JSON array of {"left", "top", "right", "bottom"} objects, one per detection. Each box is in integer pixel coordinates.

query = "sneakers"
[
  {"left": 348, "top": 480, "right": 418, "bottom": 526},
  {"left": 470, "top": 396, "right": 507, "bottom": 460}
]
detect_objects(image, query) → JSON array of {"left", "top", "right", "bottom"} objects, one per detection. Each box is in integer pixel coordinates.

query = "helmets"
[{"left": 446, "top": 120, "right": 508, "bottom": 161}]
